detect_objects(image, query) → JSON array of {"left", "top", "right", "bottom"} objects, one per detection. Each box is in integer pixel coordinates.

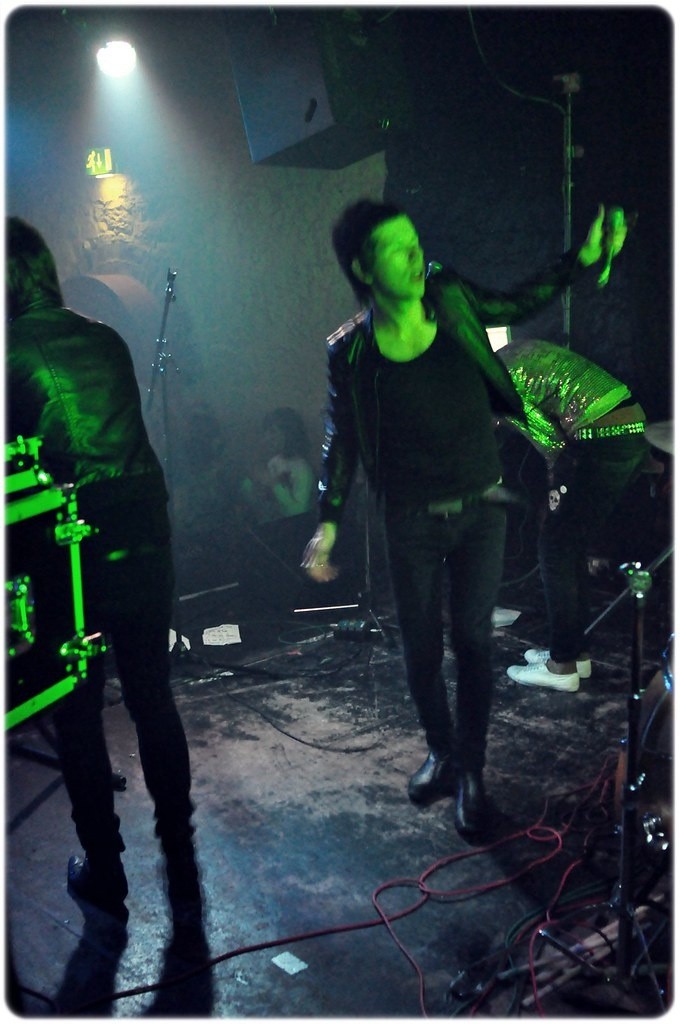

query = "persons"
[
  {"left": 6, "top": 215, "right": 202, "bottom": 907},
  {"left": 238, "top": 197, "right": 650, "bottom": 833}
]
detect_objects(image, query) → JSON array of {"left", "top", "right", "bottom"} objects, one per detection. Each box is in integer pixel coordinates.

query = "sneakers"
[
  {"left": 506, "top": 658, "right": 580, "bottom": 694},
  {"left": 524, "top": 647, "right": 593, "bottom": 679}
]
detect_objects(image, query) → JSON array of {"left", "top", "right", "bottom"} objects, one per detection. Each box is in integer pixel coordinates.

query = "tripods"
[
  {"left": 141, "top": 268, "right": 298, "bottom": 682},
  {"left": 450, "top": 541, "right": 671, "bottom": 1016},
  {"left": 289, "top": 476, "right": 397, "bottom": 647}
]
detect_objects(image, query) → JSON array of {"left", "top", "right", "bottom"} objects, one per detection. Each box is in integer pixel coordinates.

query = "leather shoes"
[
  {"left": 406, "top": 742, "right": 452, "bottom": 803},
  {"left": 64, "top": 857, "right": 128, "bottom": 911},
  {"left": 456, "top": 764, "right": 490, "bottom": 839}
]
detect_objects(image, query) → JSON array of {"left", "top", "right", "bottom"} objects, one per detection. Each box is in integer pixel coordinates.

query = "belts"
[
  {"left": 424, "top": 480, "right": 505, "bottom": 516},
  {"left": 573, "top": 421, "right": 648, "bottom": 442}
]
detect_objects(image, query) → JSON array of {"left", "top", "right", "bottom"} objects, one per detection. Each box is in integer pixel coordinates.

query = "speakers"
[{"left": 224, "top": 8, "right": 391, "bottom": 171}]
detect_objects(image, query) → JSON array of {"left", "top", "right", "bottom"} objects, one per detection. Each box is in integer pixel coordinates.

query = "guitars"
[{"left": 612, "top": 662, "right": 673, "bottom": 843}]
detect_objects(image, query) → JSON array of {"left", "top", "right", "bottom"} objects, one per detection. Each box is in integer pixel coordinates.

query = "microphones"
[{"left": 597, "top": 206, "right": 624, "bottom": 288}]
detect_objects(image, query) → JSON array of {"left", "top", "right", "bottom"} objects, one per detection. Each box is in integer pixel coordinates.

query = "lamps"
[{"left": 59, "top": 8, "right": 138, "bottom": 77}]
